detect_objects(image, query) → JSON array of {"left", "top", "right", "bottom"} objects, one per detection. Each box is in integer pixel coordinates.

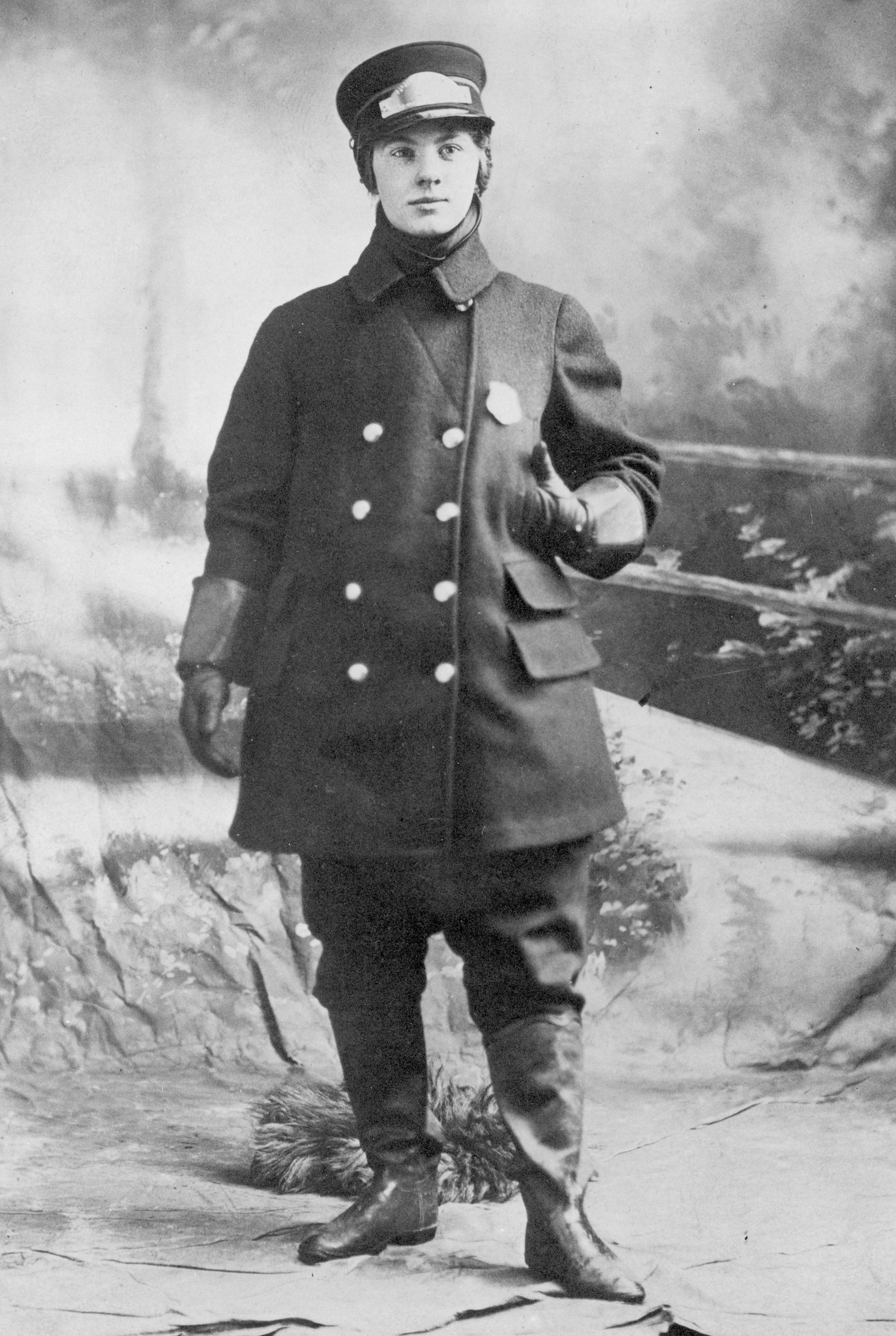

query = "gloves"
[
  {"left": 179, "top": 671, "right": 242, "bottom": 779},
  {"left": 524, "top": 443, "right": 578, "bottom": 554}
]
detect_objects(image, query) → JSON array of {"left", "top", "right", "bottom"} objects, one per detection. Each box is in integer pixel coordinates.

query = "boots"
[
  {"left": 481, "top": 1011, "right": 648, "bottom": 1306},
  {"left": 299, "top": 994, "right": 442, "bottom": 1258}
]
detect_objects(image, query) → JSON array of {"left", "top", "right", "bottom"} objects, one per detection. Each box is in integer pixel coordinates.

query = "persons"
[{"left": 175, "top": 41, "right": 665, "bottom": 1304}]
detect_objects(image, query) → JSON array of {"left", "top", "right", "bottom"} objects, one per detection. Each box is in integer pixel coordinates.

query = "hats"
[{"left": 336, "top": 39, "right": 493, "bottom": 146}]
